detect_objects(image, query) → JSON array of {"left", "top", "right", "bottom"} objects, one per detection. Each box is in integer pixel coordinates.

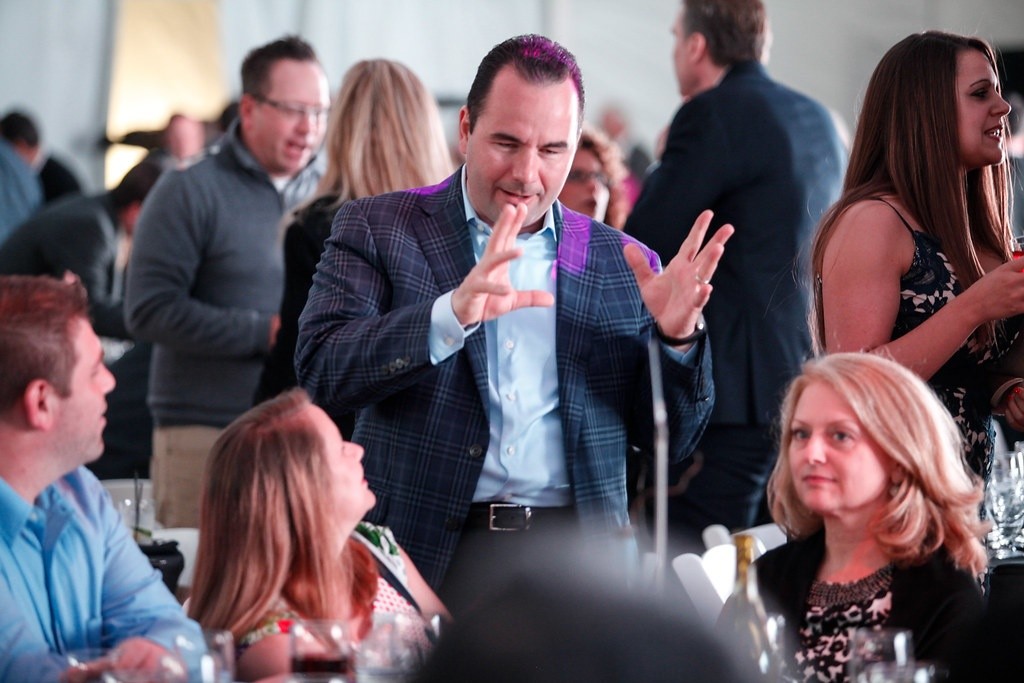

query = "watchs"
[{"left": 651, "top": 312, "right": 708, "bottom": 346}]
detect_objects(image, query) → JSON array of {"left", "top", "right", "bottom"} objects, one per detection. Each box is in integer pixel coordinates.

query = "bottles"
[
  {"left": 353, "top": 613, "right": 406, "bottom": 683},
  {"left": 718, "top": 535, "right": 776, "bottom": 683}
]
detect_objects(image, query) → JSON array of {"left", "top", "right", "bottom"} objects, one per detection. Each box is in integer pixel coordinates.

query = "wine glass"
[{"left": 987, "top": 450, "right": 1024, "bottom": 558}]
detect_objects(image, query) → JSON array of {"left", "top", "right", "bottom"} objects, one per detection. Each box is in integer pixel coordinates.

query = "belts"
[{"left": 467, "top": 503, "right": 575, "bottom": 533}]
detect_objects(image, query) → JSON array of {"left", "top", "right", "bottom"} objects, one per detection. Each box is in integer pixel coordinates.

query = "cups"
[
  {"left": 173, "top": 629, "right": 235, "bottom": 683},
  {"left": 865, "top": 662, "right": 948, "bottom": 683},
  {"left": 287, "top": 619, "right": 348, "bottom": 683},
  {"left": 1008, "top": 235, "right": 1024, "bottom": 273},
  {"left": 850, "top": 629, "right": 914, "bottom": 683}
]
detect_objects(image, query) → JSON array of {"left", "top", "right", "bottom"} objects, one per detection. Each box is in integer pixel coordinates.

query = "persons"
[
  {"left": 618, "top": 0, "right": 853, "bottom": 534},
  {"left": 293, "top": 33, "right": 734, "bottom": 594},
  {"left": 185, "top": 387, "right": 455, "bottom": 683},
  {"left": 252, "top": 57, "right": 456, "bottom": 406},
  {"left": 812, "top": 29, "right": 1024, "bottom": 498},
  {"left": 405, "top": 561, "right": 739, "bottom": 682},
  {"left": 709, "top": 351, "right": 1024, "bottom": 683},
  {"left": 556, "top": 125, "right": 627, "bottom": 232},
  {"left": 0, "top": 271, "right": 208, "bottom": 683},
  {"left": 0, "top": 102, "right": 245, "bottom": 338},
  {"left": 121, "top": 36, "right": 332, "bottom": 531}
]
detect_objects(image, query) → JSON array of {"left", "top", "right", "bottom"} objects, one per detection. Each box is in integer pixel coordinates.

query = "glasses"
[
  {"left": 251, "top": 93, "right": 333, "bottom": 123},
  {"left": 567, "top": 168, "right": 608, "bottom": 184}
]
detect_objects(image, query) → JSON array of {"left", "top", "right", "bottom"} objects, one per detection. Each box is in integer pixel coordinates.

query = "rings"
[{"left": 695, "top": 272, "right": 710, "bottom": 286}]
